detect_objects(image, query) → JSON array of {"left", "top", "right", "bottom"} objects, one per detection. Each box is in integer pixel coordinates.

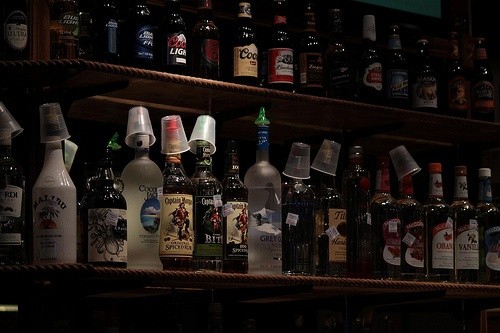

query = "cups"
[
  {"left": 125, "top": 105, "right": 217, "bottom": 156},
  {"left": 390, "top": 145, "right": 422, "bottom": 181},
  {"left": 0, "top": 101, "right": 24, "bottom": 139},
  {"left": 64, "top": 140, "right": 78, "bottom": 173},
  {"left": 281, "top": 138, "right": 341, "bottom": 180},
  {"left": 38, "top": 102, "right": 71, "bottom": 143}
]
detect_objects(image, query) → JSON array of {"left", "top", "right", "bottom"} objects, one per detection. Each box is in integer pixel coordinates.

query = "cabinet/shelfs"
[{"left": 0, "top": 0, "right": 500, "bottom": 333}]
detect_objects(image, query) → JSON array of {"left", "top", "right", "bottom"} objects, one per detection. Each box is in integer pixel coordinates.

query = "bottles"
[
  {"left": 0, "top": 0, "right": 30, "bottom": 61},
  {"left": 78, "top": 147, "right": 128, "bottom": 269},
  {"left": 191, "top": 141, "right": 223, "bottom": 275},
  {"left": 342, "top": 153, "right": 376, "bottom": 280},
  {"left": 162, "top": 152, "right": 195, "bottom": 273},
  {"left": 48, "top": 0, "right": 500, "bottom": 123},
  {"left": 220, "top": 152, "right": 251, "bottom": 275},
  {"left": 243, "top": 127, "right": 282, "bottom": 276},
  {"left": 395, "top": 159, "right": 425, "bottom": 284},
  {"left": 0, "top": 128, "right": 26, "bottom": 266},
  {"left": 450, "top": 165, "right": 478, "bottom": 284},
  {"left": 282, "top": 179, "right": 311, "bottom": 276},
  {"left": 476, "top": 167, "right": 500, "bottom": 285},
  {"left": 310, "top": 170, "right": 343, "bottom": 279},
  {"left": 371, "top": 155, "right": 401, "bottom": 282},
  {"left": 423, "top": 163, "right": 453, "bottom": 285},
  {"left": 0, "top": 287, "right": 500, "bottom": 333},
  {"left": 121, "top": 134, "right": 163, "bottom": 272},
  {"left": 30, "top": 106, "right": 78, "bottom": 266}
]
